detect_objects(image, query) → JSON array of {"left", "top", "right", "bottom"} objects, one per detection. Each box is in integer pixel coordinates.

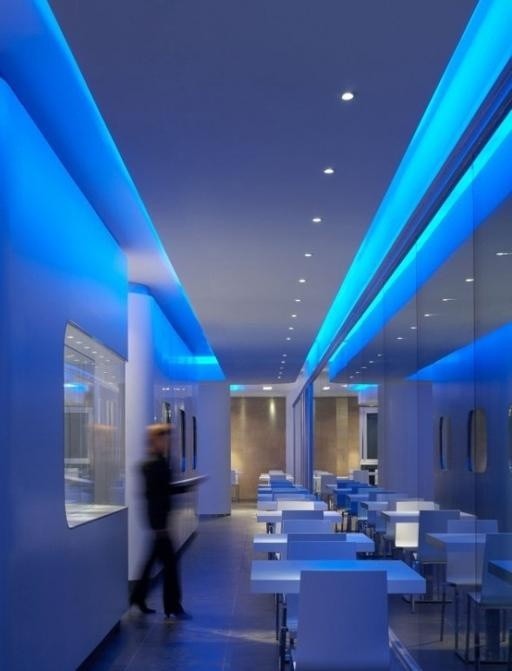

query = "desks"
[{"left": 247, "top": 475, "right": 428, "bottom": 670}]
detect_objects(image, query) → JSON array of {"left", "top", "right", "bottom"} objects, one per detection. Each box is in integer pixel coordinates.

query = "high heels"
[
  {"left": 164, "top": 606, "right": 193, "bottom": 619},
  {"left": 129, "top": 591, "right": 156, "bottom": 614}
]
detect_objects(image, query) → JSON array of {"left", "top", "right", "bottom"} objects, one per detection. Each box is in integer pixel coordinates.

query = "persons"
[{"left": 127, "top": 420, "right": 194, "bottom": 621}]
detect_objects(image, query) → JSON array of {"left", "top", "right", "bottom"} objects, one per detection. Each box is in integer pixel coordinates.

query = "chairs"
[
  {"left": 268, "top": 473, "right": 390, "bottom": 671},
  {"left": 312, "top": 472, "right": 511, "bottom": 671}
]
